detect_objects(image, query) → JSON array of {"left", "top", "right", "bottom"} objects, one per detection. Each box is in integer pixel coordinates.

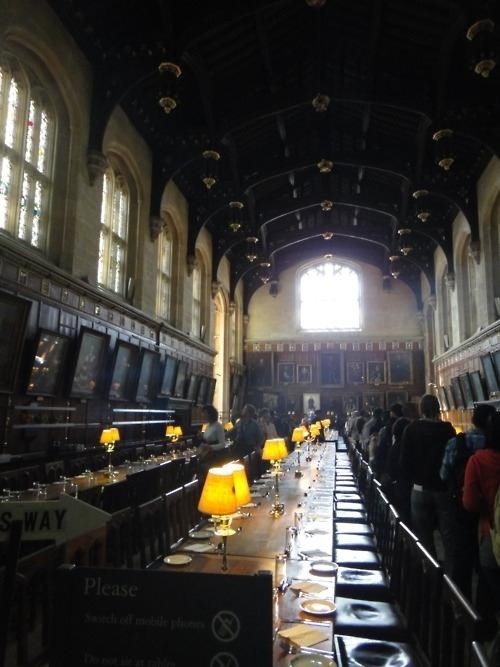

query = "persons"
[
  {"left": 336, "top": 393, "right": 500, "bottom": 667},
  {"left": 251, "top": 355, "right": 404, "bottom": 412},
  {"left": 191, "top": 401, "right": 332, "bottom": 524}
]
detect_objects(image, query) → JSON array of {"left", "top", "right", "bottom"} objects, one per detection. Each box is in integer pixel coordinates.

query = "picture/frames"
[
  {"left": 23, "top": 325, "right": 217, "bottom": 408},
  {"left": 431, "top": 349, "right": 500, "bottom": 413},
  {"left": 246, "top": 349, "right": 414, "bottom": 416}
]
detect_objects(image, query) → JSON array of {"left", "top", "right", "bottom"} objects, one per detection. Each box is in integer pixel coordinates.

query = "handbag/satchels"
[{"left": 198, "top": 445, "right": 214, "bottom": 461}]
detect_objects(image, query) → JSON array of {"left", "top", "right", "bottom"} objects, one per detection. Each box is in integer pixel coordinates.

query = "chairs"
[{"left": 0, "top": 431, "right": 488, "bottom": 667}]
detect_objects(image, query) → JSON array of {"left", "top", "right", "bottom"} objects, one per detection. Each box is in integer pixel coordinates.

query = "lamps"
[
  {"left": 203, "top": 150, "right": 220, "bottom": 190},
  {"left": 433, "top": 131, "right": 453, "bottom": 170},
  {"left": 464, "top": 17, "right": 499, "bottom": 78},
  {"left": 300, "top": 418, "right": 330, "bottom": 446},
  {"left": 413, "top": 188, "right": 430, "bottom": 223},
  {"left": 157, "top": 62, "right": 182, "bottom": 114},
  {"left": 244, "top": 227, "right": 412, "bottom": 286},
  {"left": 229, "top": 201, "right": 244, "bottom": 231},
  {"left": 166, "top": 425, "right": 182, "bottom": 458},
  {"left": 201, "top": 423, "right": 210, "bottom": 433},
  {"left": 225, "top": 422, "right": 233, "bottom": 432}
]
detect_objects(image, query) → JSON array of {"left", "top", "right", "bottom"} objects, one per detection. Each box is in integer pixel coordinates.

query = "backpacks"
[
  {"left": 487, "top": 485, "right": 500, "bottom": 568},
  {"left": 454, "top": 432, "right": 470, "bottom": 493}
]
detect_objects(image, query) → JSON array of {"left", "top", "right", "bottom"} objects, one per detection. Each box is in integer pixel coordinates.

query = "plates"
[
  {"left": 289, "top": 559, "right": 339, "bottom": 667},
  {"left": 163, "top": 469, "right": 272, "bottom": 565}
]
